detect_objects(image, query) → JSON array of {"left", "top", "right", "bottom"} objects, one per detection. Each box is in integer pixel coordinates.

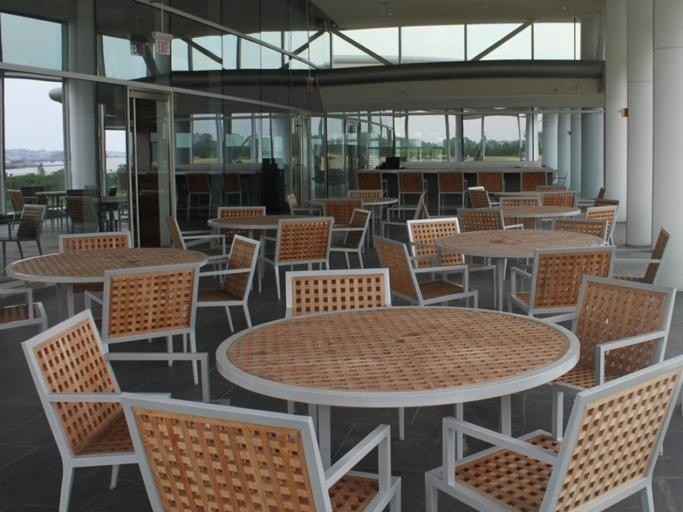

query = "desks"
[
  {"left": 204, "top": 215, "right": 283, "bottom": 232},
  {"left": 213, "top": 305, "right": 580, "bottom": 511},
  {"left": 34, "top": 190, "right": 74, "bottom": 229},
  {"left": 490, "top": 205, "right": 583, "bottom": 217},
  {"left": 433, "top": 228, "right": 612, "bottom": 313},
  {"left": 4, "top": 247, "right": 229, "bottom": 333}
]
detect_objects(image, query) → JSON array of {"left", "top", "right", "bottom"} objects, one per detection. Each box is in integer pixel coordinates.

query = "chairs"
[
  {"left": 434, "top": 168, "right": 467, "bottom": 214},
  {"left": 582, "top": 205, "right": 622, "bottom": 245},
  {"left": 166, "top": 208, "right": 238, "bottom": 275},
  {"left": 326, "top": 208, "right": 373, "bottom": 276},
  {"left": 466, "top": 186, "right": 496, "bottom": 214},
  {"left": 518, "top": 165, "right": 550, "bottom": 198},
  {"left": 454, "top": 208, "right": 523, "bottom": 235},
  {"left": 537, "top": 190, "right": 576, "bottom": 211},
  {"left": 285, "top": 195, "right": 319, "bottom": 217},
  {"left": 342, "top": 188, "right": 388, "bottom": 206},
  {"left": 610, "top": 228, "right": 670, "bottom": 289},
  {"left": 425, "top": 351, "right": 683, "bottom": 510},
  {"left": 61, "top": 198, "right": 106, "bottom": 237},
  {"left": 0, "top": 272, "right": 52, "bottom": 342},
  {"left": 406, "top": 217, "right": 502, "bottom": 306},
  {"left": 549, "top": 216, "right": 610, "bottom": 255},
  {"left": 82, "top": 262, "right": 204, "bottom": 387},
  {"left": 383, "top": 192, "right": 428, "bottom": 229},
  {"left": 178, "top": 235, "right": 265, "bottom": 331},
  {"left": 371, "top": 233, "right": 478, "bottom": 316},
  {"left": 396, "top": 169, "right": 429, "bottom": 207},
  {"left": 19, "top": 308, "right": 232, "bottom": 512},
  {"left": 183, "top": 174, "right": 211, "bottom": 209},
  {"left": 219, "top": 174, "right": 250, "bottom": 208},
  {"left": 475, "top": 169, "right": 507, "bottom": 199},
  {"left": 318, "top": 197, "right": 360, "bottom": 212},
  {"left": 546, "top": 276, "right": 678, "bottom": 457},
  {"left": 117, "top": 391, "right": 402, "bottom": 507},
  {"left": 258, "top": 216, "right": 336, "bottom": 302},
  {"left": 351, "top": 168, "right": 388, "bottom": 202},
  {"left": 55, "top": 231, "right": 137, "bottom": 260},
  {"left": 283, "top": 266, "right": 399, "bottom": 438},
  {"left": 506, "top": 244, "right": 625, "bottom": 320},
  {"left": 2, "top": 203, "right": 46, "bottom": 257}
]
